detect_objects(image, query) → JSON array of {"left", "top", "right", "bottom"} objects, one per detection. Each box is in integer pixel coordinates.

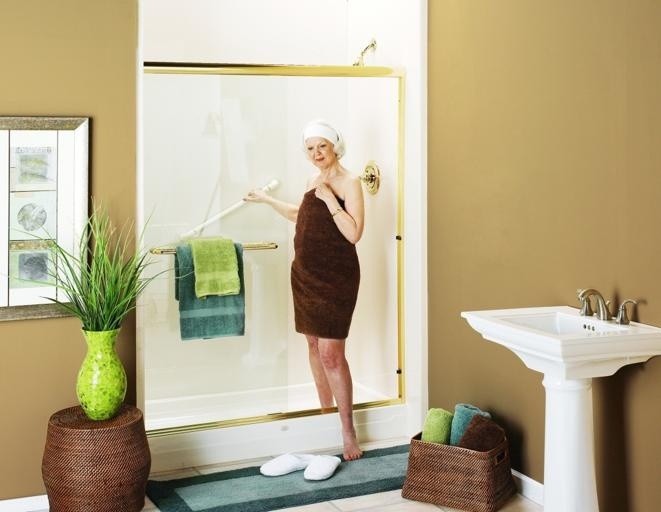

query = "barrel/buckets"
[
  {"left": 304, "top": 453, "right": 342, "bottom": 480},
  {"left": 260, "top": 451, "right": 320, "bottom": 477}
]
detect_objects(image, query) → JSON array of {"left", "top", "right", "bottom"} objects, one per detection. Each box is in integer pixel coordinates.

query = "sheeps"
[{"left": 578, "top": 289, "right": 611, "bottom": 320}]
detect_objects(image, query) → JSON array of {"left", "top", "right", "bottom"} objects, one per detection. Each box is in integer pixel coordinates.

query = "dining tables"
[{"left": 35, "top": 213, "right": 173, "bottom": 425}]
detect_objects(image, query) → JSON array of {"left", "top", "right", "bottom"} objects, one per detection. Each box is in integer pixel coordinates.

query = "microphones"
[{"left": 38, "top": 400, "right": 153, "bottom": 512}]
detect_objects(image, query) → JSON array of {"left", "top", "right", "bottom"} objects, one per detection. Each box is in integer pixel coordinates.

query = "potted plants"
[{"left": 0, "top": 112, "right": 94, "bottom": 323}]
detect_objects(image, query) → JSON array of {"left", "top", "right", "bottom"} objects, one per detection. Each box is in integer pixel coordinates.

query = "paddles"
[{"left": 143, "top": 438, "right": 411, "bottom": 512}]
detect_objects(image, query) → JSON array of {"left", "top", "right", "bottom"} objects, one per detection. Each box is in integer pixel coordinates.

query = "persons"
[{"left": 243, "top": 119, "right": 366, "bottom": 463}]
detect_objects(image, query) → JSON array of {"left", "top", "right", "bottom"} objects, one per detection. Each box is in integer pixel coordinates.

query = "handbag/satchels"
[{"left": 331, "top": 208, "right": 347, "bottom": 217}]
